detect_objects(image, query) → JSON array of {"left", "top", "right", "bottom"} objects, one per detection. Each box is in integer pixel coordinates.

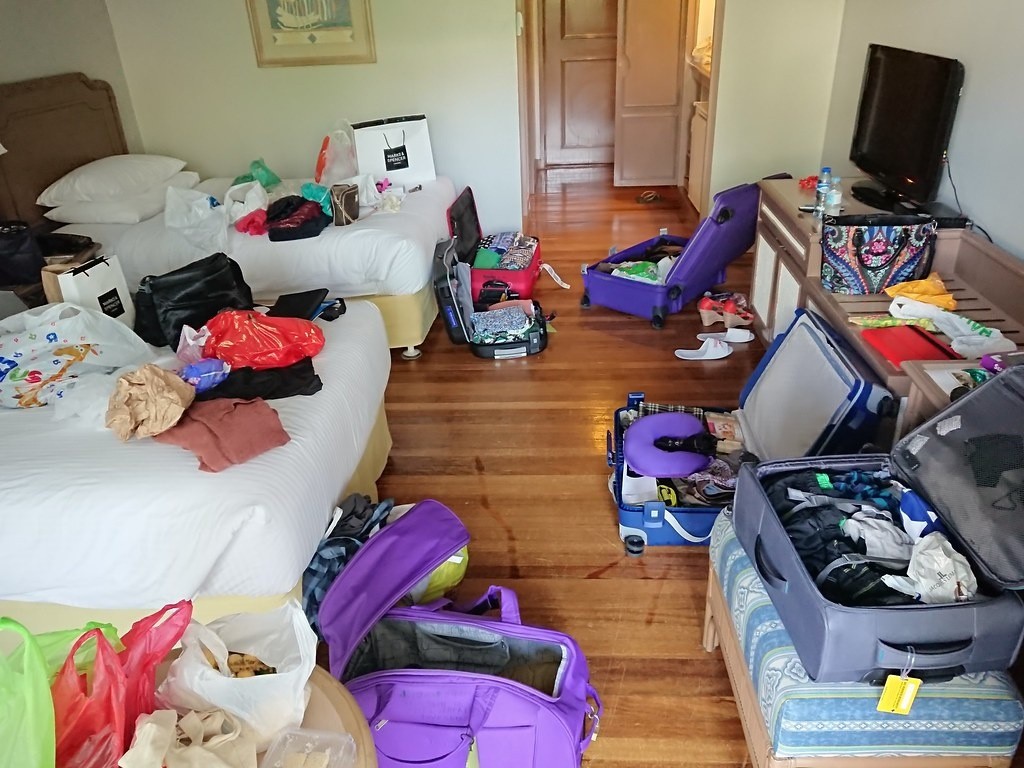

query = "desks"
[{"left": 152, "top": 647, "right": 378, "bottom": 768}]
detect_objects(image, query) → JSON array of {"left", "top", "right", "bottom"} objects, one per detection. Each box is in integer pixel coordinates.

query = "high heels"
[
  {"left": 699, "top": 297, "right": 724, "bottom": 326},
  {"left": 722, "top": 300, "right": 754, "bottom": 329}
]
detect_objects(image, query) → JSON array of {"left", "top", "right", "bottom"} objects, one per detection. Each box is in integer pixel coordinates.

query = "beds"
[
  {"left": 0, "top": 298, "right": 394, "bottom": 658},
  {"left": 0, "top": 72, "right": 458, "bottom": 360}
]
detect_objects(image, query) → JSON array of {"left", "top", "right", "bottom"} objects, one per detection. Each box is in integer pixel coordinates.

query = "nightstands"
[{"left": 64, "top": 239, "right": 103, "bottom": 267}]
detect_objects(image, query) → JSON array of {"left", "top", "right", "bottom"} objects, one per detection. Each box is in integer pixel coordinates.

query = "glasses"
[{"left": 320, "top": 298, "right": 347, "bottom": 322}]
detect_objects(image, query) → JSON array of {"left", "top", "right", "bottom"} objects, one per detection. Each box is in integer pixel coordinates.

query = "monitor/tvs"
[{"left": 848, "top": 43, "right": 964, "bottom": 211}]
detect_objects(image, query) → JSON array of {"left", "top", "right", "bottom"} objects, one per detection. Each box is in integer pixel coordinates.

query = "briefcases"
[
  {"left": 734, "top": 377, "right": 1023, "bottom": 686},
  {"left": 434, "top": 236, "right": 556, "bottom": 360}
]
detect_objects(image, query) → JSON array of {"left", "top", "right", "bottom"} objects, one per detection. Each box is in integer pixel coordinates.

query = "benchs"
[{"left": 701, "top": 508, "right": 1024, "bottom": 768}]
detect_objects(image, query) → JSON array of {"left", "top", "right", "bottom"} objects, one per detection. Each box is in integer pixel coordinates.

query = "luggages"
[
  {"left": 606, "top": 308, "right": 874, "bottom": 557},
  {"left": 579, "top": 173, "right": 793, "bottom": 329},
  {"left": 446, "top": 186, "right": 543, "bottom": 306}
]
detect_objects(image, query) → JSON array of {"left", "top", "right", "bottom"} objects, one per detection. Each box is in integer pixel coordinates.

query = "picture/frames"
[{"left": 245, "top": 0, "right": 377, "bottom": 68}]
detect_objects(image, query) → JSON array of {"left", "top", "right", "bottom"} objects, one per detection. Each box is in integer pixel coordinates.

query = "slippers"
[
  {"left": 698, "top": 328, "right": 755, "bottom": 343},
  {"left": 636, "top": 191, "right": 664, "bottom": 203},
  {"left": 675, "top": 338, "right": 733, "bottom": 360}
]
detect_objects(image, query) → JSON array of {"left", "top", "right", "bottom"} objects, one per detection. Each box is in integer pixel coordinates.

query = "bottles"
[{"left": 814, "top": 167, "right": 841, "bottom": 217}]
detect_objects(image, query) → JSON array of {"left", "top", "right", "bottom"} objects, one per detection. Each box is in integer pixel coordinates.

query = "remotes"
[{"left": 799, "top": 204, "right": 846, "bottom": 212}]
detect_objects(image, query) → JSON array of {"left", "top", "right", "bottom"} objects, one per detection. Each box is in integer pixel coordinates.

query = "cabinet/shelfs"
[
  {"left": 749, "top": 175, "right": 1024, "bottom": 444},
  {"left": 611, "top": 0, "right": 716, "bottom": 212}
]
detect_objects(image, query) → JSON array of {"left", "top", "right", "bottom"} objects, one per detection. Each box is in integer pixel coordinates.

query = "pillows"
[
  {"left": 43, "top": 171, "right": 200, "bottom": 225},
  {"left": 35, "top": 154, "right": 187, "bottom": 207}
]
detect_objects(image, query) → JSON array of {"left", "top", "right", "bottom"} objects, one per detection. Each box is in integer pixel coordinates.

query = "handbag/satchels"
[
  {"left": 0, "top": 219, "right": 47, "bottom": 287},
  {"left": 317, "top": 499, "right": 601, "bottom": 768},
  {"left": 0, "top": 253, "right": 329, "bottom": 416},
  {"left": 0, "top": 598, "right": 320, "bottom": 768},
  {"left": 887, "top": 295, "right": 1017, "bottom": 360},
  {"left": 162, "top": 120, "right": 360, "bottom": 247},
  {"left": 303, "top": 495, "right": 393, "bottom": 630},
  {"left": 351, "top": 114, "right": 436, "bottom": 189},
  {"left": 822, "top": 213, "right": 936, "bottom": 295}
]
